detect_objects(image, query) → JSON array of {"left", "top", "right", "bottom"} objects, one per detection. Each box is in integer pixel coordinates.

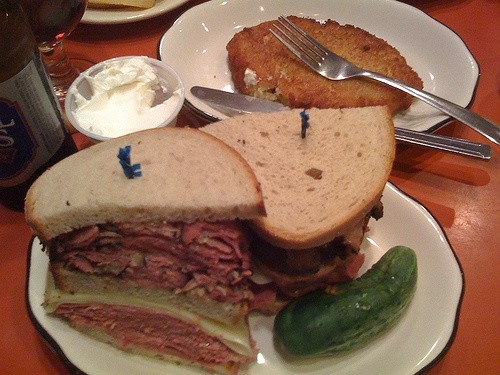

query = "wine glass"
[{"left": 21, "top": 0, "right": 99, "bottom": 116}]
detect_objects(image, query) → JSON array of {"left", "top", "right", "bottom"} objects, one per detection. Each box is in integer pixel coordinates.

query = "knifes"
[{"left": 190, "top": 86, "right": 491, "bottom": 160}]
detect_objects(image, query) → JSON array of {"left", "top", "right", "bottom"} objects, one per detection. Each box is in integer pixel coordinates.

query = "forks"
[{"left": 268, "top": 15, "right": 500, "bottom": 146}]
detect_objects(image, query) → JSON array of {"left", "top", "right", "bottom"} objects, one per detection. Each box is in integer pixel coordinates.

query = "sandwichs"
[
  {"left": 199, "top": 104, "right": 396, "bottom": 302},
  {"left": 23, "top": 127, "right": 266, "bottom": 375}
]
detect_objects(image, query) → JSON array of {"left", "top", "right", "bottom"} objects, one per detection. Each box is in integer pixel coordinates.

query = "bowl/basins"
[{"left": 63, "top": 55, "right": 185, "bottom": 142}]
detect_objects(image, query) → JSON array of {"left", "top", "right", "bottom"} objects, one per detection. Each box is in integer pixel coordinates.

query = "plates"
[
  {"left": 25, "top": 178, "right": 465, "bottom": 375},
  {"left": 81, "top": 0, "right": 190, "bottom": 26},
  {"left": 157, "top": 0, "right": 480, "bottom": 146}
]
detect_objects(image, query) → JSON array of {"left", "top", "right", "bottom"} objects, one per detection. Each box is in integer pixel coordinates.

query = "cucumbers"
[{"left": 275, "top": 246, "right": 418, "bottom": 356}]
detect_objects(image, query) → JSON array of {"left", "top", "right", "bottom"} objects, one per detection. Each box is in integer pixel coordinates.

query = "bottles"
[{"left": 0, "top": 0, "right": 78, "bottom": 211}]
